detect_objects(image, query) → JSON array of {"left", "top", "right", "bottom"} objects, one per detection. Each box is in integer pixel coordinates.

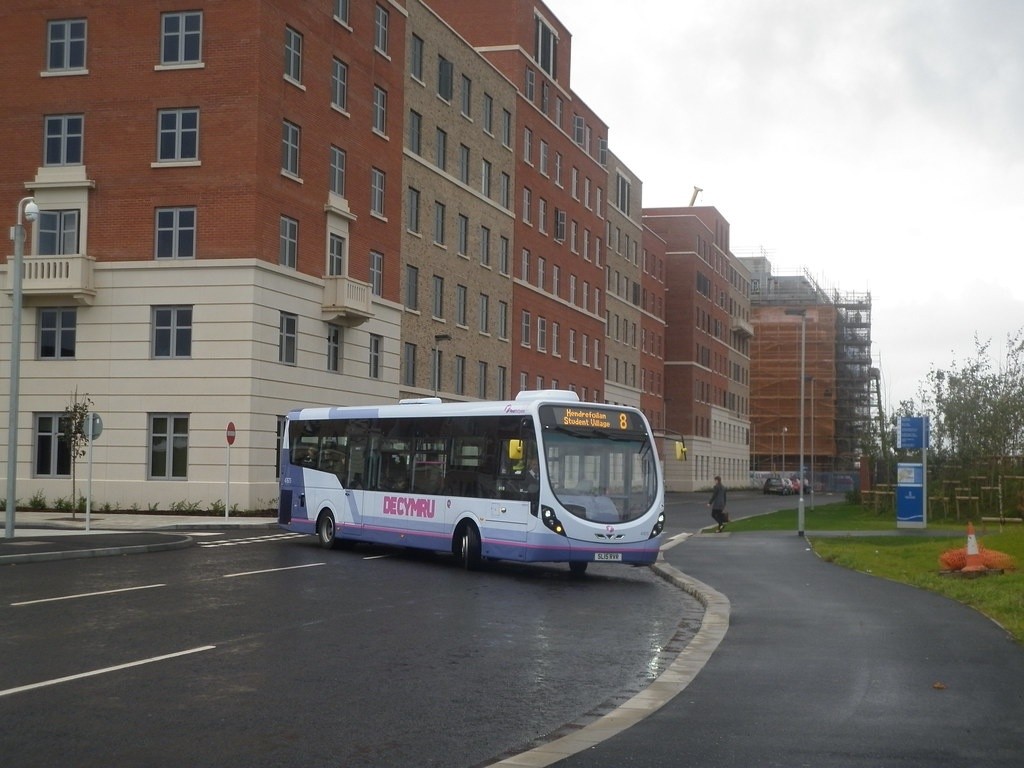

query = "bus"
[{"left": 277, "top": 389, "right": 687, "bottom": 570}]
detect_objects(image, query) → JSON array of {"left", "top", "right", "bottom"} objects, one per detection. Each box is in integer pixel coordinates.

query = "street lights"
[
  {"left": 784, "top": 306, "right": 806, "bottom": 537},
  {"left": 3, "top": 196, "right": 41, "bottom": 539}
]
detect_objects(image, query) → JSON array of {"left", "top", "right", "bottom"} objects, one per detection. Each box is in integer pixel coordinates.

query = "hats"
[{"left": 715, "top": 476, "right": 721, "bottom": 481}]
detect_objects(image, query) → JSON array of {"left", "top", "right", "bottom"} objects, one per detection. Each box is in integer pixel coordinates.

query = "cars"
[{"left": 764, "top": 476, "right": 824, "bottom": 495}]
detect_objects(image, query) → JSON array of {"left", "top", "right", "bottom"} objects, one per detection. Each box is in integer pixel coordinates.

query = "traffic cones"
[{"left": 960, "top": 520, "right": 990, "bottom": 574}]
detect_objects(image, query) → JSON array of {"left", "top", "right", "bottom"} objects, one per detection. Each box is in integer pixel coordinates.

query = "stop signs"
[{"left": 227, "top": 421, "right": 236, "bottom": 445}]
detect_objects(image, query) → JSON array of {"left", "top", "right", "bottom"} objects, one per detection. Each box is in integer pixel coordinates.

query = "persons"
[
  {"left": 708, "top": 476, "right": 726, "bottom": 532},
  {"left": 529, "top": 459, "right": 558, "bottom": 488},
  {"left": 304, "top": 447, "right": 315, "bottom": 463}
]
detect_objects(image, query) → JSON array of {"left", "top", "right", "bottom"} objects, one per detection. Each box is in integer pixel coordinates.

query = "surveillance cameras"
[{"left": 25, "top": 203, "right": 38, "bottom": 222}]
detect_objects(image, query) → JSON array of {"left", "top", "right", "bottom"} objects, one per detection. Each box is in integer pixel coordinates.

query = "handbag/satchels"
[{"left": 721, "top": 512, "right": 728, "bottom": 522}]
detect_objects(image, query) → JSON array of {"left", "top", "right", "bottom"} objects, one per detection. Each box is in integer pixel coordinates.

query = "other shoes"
[
  {"left": 715, "top": 529, "right": 721, "bottom": 533},
  {"left": 720, "top": 525, "right": 724, "bottom": 531}
]
